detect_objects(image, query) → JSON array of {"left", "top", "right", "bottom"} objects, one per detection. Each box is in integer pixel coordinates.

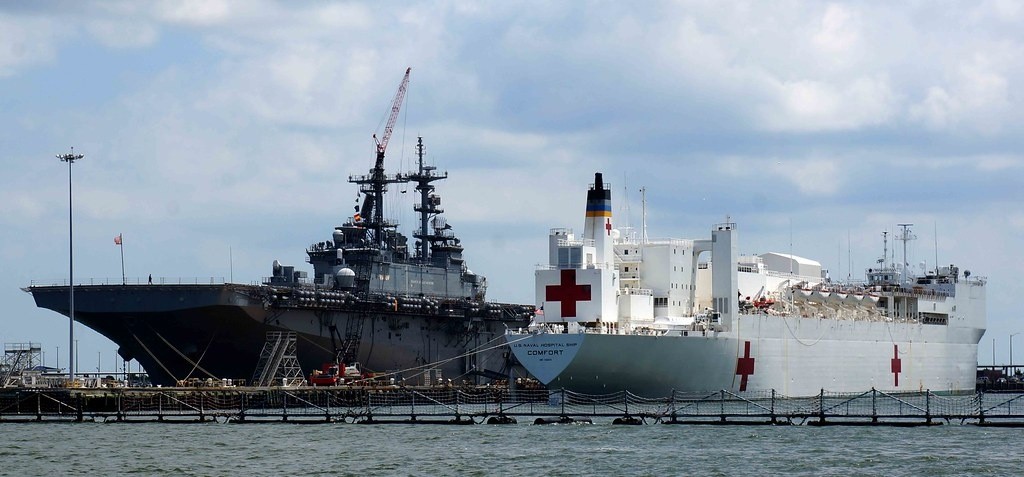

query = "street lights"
[
  {"left": 1010, "top": 332, "right": 1021, "bottom": 376},
  {"left": 54, "top": 145, "right": 86, "bottom": 387}
]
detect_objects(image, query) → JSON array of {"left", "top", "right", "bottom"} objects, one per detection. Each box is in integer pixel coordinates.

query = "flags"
[{"left": 114, "top": 235, "right": 122, "bottom": 245}]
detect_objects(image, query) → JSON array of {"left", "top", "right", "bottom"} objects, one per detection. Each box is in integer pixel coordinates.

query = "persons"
[
  {"left": 148, "top": 274, "right": 152, "bottom": 285},
  {"left": 388, "top": 375, "right": 542, "bottom": 387}
]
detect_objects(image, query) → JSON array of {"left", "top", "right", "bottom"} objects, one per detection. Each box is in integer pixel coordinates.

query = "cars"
[{"left": 997, "top": 377, "right": 1006, "bottom": 383}]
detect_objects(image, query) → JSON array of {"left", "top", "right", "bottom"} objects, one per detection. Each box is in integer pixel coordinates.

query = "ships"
[
  {"left": 19, "top": 134, "right": 535, "bottom": 406},
  {"left": 500, "top": 170, "right": 990, "bottom": 403}
]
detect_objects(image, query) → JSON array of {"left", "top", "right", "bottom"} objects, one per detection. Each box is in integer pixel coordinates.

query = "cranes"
[{"left": 353, "top": 65, "right": 412, "bottom": 221}]
